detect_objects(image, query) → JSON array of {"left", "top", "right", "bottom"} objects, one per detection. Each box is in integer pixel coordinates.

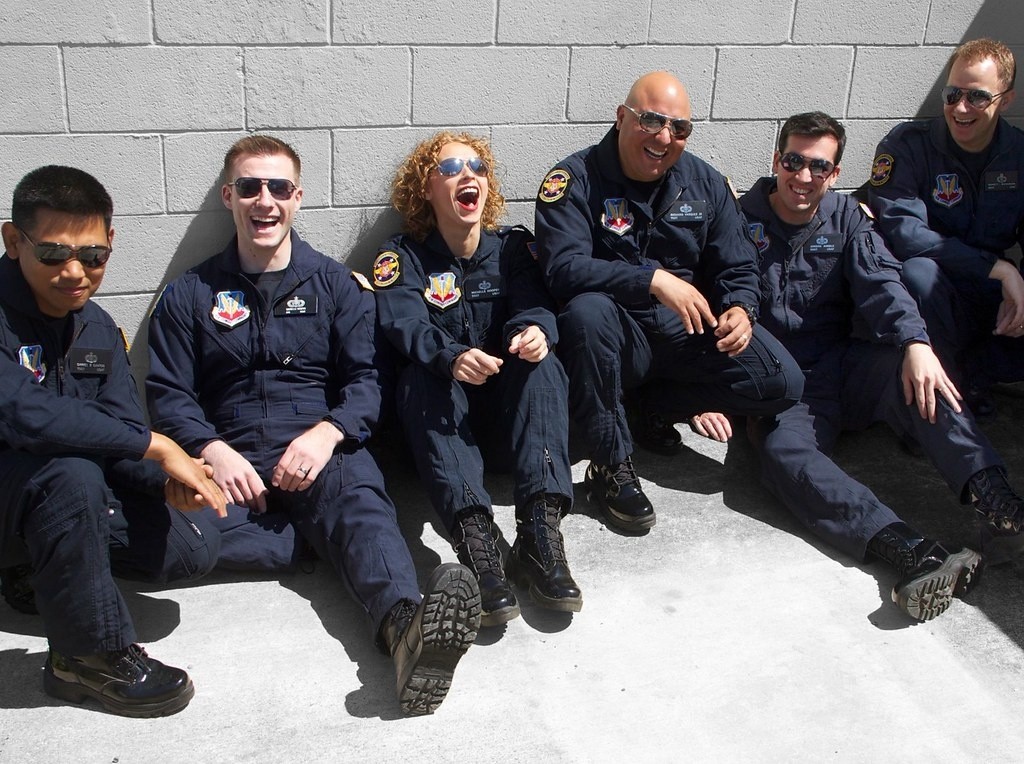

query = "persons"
[
  {"left": 735, "top": 110, "right": 1023, "bottom": 621},
  {"left": 369, "top": 132, "right": 583, "bottom": 629},
  {"left": 867, "top": 38, "right": 1024, "bottom": 458},
  {"left": 143, "top": 137, "right": 483, "bottom": 717},
  {"left": 0, "top": 164, "right": 228, "bottom": 717},
  {"left": 534, "top": 67, "right": 806, "bottom": 533}
]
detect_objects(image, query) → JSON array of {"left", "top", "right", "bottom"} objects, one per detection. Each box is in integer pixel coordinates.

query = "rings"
[
  {"left": 939, "top": 385, "right": 946, "bottom": 391},
  {"left": 742, "top": 334, "right": 748, "bottom": 341},
  {"left": 1019, "top": 325, "right": 1023, "bottom": 329},
  {"left": 298, "top": 467, "right": 307, "bottom": 474}
]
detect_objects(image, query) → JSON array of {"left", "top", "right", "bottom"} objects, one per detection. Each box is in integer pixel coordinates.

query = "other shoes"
[{"left": 960, "top": 376, "right": 993, "bottom": 413}]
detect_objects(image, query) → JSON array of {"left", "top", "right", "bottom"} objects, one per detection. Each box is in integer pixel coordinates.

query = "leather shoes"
[
  {"left": 0, "top": 565, "right": 41, "bottom": 615},
  {"left": 45, "top": 648, "right": 194, "bottom": 718},
  {"left": 627, "top": 402, "right": 684, "bottom": 452},
  {"left": 582, "top": 456, "right": 655, "bottom": 533}
]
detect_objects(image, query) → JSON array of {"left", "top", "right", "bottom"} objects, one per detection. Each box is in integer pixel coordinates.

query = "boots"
[
  {"left": 869, "top": 523, "right": 983, "bottom": 622},
  {"left": 968, "top": 465, "right": 1024, "bottom": 566},
  {"left": 504, "top": 493, "right": 582, "bottom": 612},
  {"left": 448, "top": 505, "right": 519, "bottom": 627},
  {"left": 379, "top": 564, "right": 481, "bottom": 716}
]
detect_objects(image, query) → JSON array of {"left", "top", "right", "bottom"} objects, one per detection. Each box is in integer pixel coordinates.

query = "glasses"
[
  {"left": 432, "top": 158, "right": 489, "bottom": 177},
  {"left": 228, "top": 178, "right": 297, "bottom": 200},
  {"left": 941, "top": 86, "right": 1010, "bottom": 108},
  {"left": 778, "top": 153, "right": 835, "bottom": 183},
  {"left": 622, "top": 104, "right": 693, "bottom": 140},
  {"left": 13, "top": 225, "right": 112, "bottom": 268}
]
